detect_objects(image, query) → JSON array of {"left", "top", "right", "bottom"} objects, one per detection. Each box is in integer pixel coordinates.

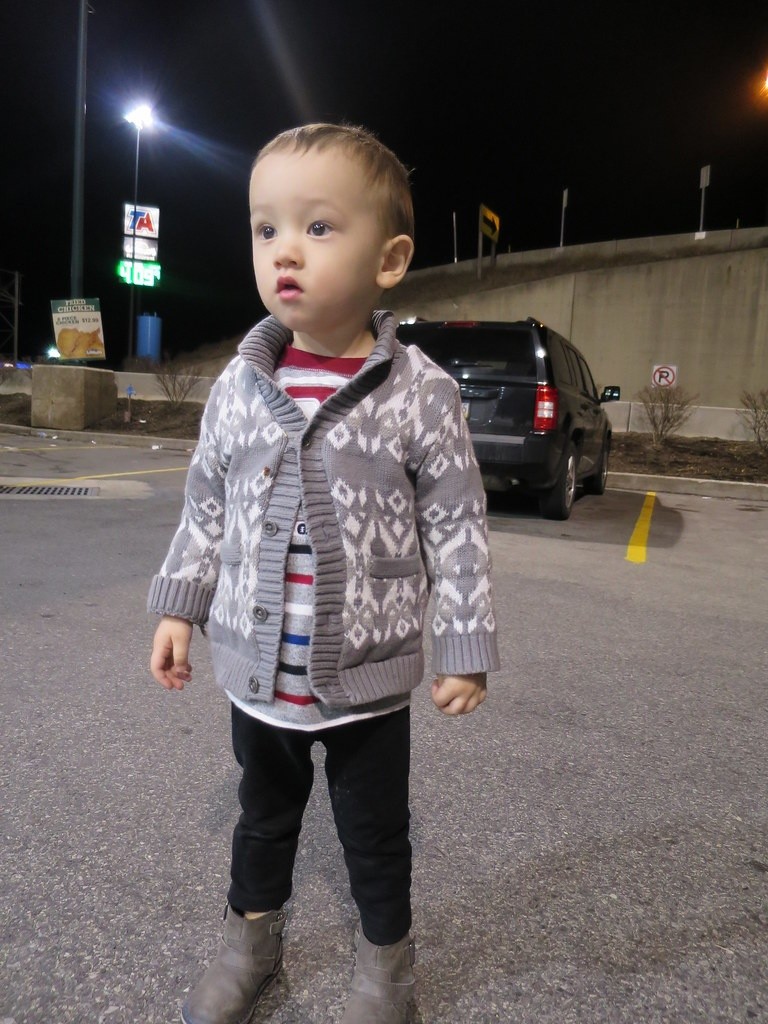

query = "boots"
[
  {"left": 343, "top": 915, "right": 417, "bottom": 1024},
  {"left": 180, "top": 901, "right": 288, "bottom": 1024}
]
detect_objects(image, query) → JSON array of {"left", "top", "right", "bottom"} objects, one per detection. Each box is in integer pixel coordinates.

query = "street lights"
[{"left": 125, "top": 105, "right": 151, "bottom": 373}]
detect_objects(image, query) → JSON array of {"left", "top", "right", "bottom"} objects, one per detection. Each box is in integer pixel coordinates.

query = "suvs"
[{"left": 396, "top": 316, "right": 624, "bottom": 522}]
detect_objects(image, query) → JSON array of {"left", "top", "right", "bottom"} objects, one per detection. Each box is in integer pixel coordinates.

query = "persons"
[{"left": 146, "top": 123, "right": 499, "bottom": 1024}]
